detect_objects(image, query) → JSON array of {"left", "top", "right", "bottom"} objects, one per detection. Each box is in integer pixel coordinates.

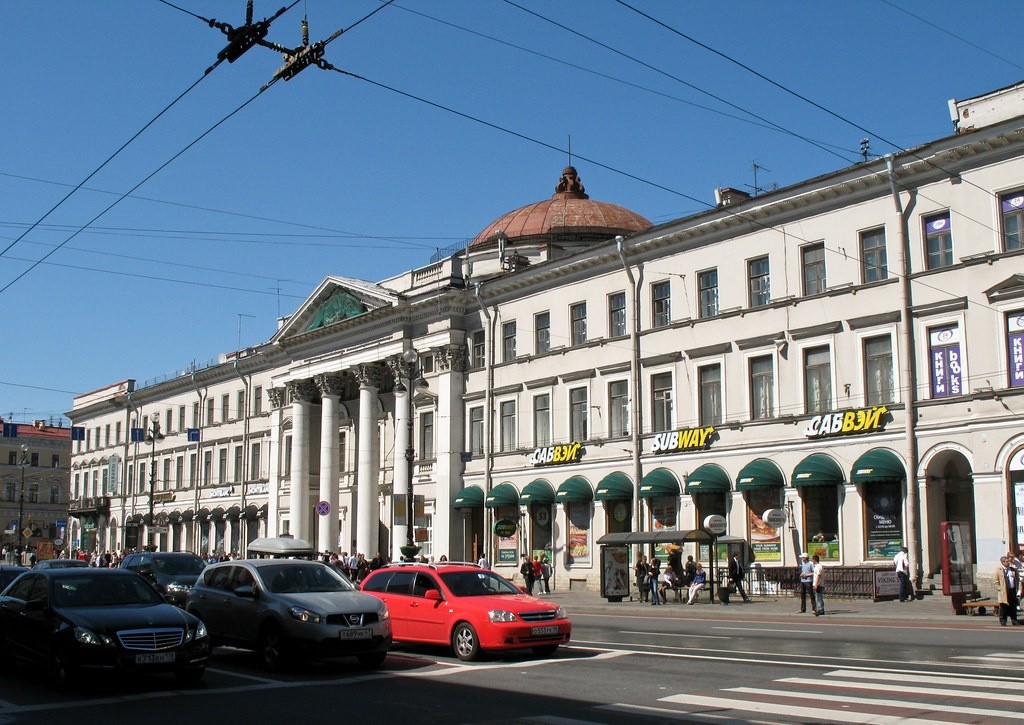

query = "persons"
[
  {"left": 726, "top": 554, "right": 752, "bottom": 603},
  {"left": 894, "top": 547, "right": 908, "bottom": 602},
  {"left": 418, "top": 555, "right": 447, "bottom": 563},
  {"left": 798, "top": 553, "right": 825, "bottom": 616},
  {"left": 520, "top": 556, "right": 551, "bottom": 595},
  {"left": 659, "top": 565, "right": 676, "bottom": 604},
  {"left": 317, "top": 549, "right": 404, "bottom": 582},
  {"left": 478, "top": 553, "right": 489, "bottom": 585},
  {"left": 202, "top": 550, "right": 239, "bottom": 564},
  {"left": 993, "top": 552, "right": 1024, "bottom": 626},
  {"left": 687, "top": 564, "right": 706, "bottom": 605},
  {"left": 2, "top": 542, "right": 127, "bottom": 569},
  {"left": 668, "top": 549, "right": 697, "bottom": 587},
  {"left": 635, "top": 553, "right": 660, "bottom": 605}
]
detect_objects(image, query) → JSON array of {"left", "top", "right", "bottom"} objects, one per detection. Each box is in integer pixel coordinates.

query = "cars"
[
  {"left": 185, "top": 559, "right": 393, "bottom": 677},
  {"left": 32, "top": 559, "right": 99, "bottom": 598},
  {"left": 360, "top": 560, "right": 572, "bottom": 662},
  {"left": 120, "top": 552, "right": 228, "bottom": 612},
  {"left": 0, "top": 565, "right": 31, "bottom": 595},
  {"left": 0, "top": 567, "right": 210, "bottom": 697}
]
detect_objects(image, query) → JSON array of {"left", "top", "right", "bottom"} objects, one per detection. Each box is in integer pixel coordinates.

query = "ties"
[{"left": 1005, "top": 568, "right": 1012, "bottom": 588}]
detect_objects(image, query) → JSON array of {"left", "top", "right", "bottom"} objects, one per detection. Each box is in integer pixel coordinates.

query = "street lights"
[
  {"left": 143, "top": 412, "right": 164, "bottom": 552},
  {"left": 392, "top": 348, "right": 429, "bottom": 563},
  {"left": 17, "top": 443, "right": 31, "bottom": 565}
]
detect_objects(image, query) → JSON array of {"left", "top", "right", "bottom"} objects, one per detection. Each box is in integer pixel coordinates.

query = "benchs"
[{"left": 657, "top": 587, "right": 704, "bottom": 603}]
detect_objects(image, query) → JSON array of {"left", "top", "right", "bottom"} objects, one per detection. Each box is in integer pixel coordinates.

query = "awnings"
[
  {"left": 736, "top": 459, "right": 785, "bottom": 490},
  {"left": 454, "top": 464, "right": 731, "bottom": 510},
  {"left": 852, "top": 449, "right": 905, "bottom": 483},
  {"left": 791, "top": 453, "right": 843, "bottom": 487}
]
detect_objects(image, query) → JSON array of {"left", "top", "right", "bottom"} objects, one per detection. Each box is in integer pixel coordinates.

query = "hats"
[{"left": 799, "top": 552, "right": 808, "bottom": 558}]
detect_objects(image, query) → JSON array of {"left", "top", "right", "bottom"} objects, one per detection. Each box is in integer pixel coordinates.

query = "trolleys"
[{"left": 961, "top": 601, "right": 1001, "bottom": 617}]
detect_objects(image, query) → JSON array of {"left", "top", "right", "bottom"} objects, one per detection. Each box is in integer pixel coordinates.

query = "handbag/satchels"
[{"left": 643, "top": 575, "right": 650, "bottom": 584}]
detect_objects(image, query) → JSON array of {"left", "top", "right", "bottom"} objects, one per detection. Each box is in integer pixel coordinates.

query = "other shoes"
[
  {"left": 663, "top": 599, "right": 667, "bottom": 604},
  {"left": 812, "top": 610, "right": 816, "bottom": 614},
  {"left": 538, "top": 592, "right": 543, "bottom": 595},
  {"left": 796, "top": 610, "right": 804, "bottom": 613},
  {"left": 744, "top": 597, "right": 752, "bottom": 603},
  {"left": 547, "top": 591, "right": 551, "bottom": 595},
  {"left": 651, "top": 603, "right": 656, "bottom": 605},
  {"left": 687, "top": 602, "right": 692, "bottom": 605},
  {"left": 543, "top": 591, "right": 547, "bottom": 594}
]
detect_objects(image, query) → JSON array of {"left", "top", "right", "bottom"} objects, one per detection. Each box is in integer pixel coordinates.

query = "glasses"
[{"left": 1004, "top": 560, "right": 1010, "bottom": 562}]
